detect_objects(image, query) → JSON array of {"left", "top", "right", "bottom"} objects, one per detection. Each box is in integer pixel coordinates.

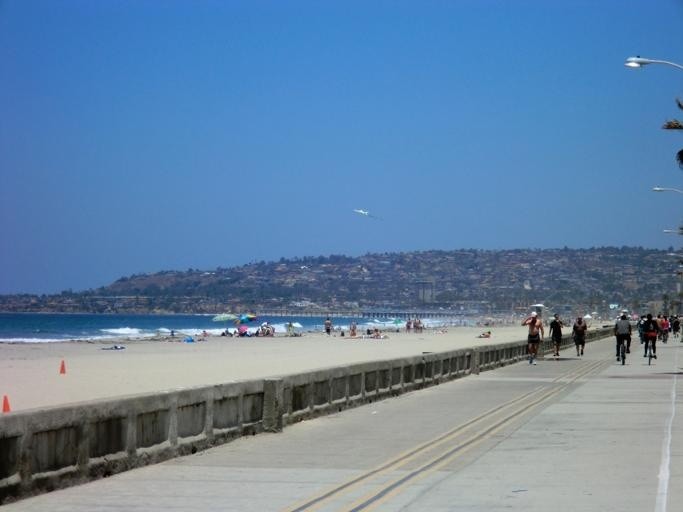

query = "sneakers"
[{"left": 528, "top": 336, "right": 683, "bottom": 365}]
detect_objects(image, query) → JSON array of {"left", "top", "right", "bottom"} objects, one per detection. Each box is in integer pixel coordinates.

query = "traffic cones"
[
  {"left": 3, "top": 395, "right": 11, "bottom": 413},
  {"left": 59, "top": 360, "right": 66, "bottom": 375}
]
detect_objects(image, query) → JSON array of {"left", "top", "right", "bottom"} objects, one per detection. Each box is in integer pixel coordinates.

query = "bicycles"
[
  {"left": 644, "top": 338, "right": 657, "bottom": 366},
  {"left": 614, "top": 333, "right": 633, "bottom": 365}
]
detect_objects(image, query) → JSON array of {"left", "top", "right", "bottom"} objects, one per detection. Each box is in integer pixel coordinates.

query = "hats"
[{"left": 530, "top": 311, "right": 538, "bottom": 318}]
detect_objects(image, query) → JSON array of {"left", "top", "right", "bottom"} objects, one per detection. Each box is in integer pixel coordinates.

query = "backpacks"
[{"left": 644, "top": 322, "right": 653, "bottom": 331}]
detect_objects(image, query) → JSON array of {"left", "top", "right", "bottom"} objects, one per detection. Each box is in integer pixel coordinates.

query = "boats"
[{"left": 212, "top": 311, "right": 257, "bottom": 322}]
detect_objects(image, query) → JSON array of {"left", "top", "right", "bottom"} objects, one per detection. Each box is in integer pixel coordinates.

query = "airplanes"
[{"left": 353, "top": 208, "right": 368, "bottom": 217}]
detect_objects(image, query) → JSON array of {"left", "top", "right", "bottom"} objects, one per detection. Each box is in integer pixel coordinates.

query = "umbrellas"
[
  {"left": 234, "top": 312, "right": 257, "bottom": 325},
  {"left": 212, "top": 311, "right": 237, "bottom": 331}
]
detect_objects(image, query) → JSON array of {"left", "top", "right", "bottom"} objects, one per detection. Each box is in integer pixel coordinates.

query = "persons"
[
  {"left": 613, "top": 312, "right": 681, "bottom": 344},
  {"left": 548, "top": 313, "right": 564, "bottom": 357},
  {"left": 571, "top": 316, "right": 588, "bottom": 356},
  {"left": 643, "top": 314, "right": 661, "bottom": 359},
  {"left": 521, "top": 311, "right": 546, "bottom": 366},
  {"left": 614, "top": 314, "right": 632, "bottom": 361}
]
